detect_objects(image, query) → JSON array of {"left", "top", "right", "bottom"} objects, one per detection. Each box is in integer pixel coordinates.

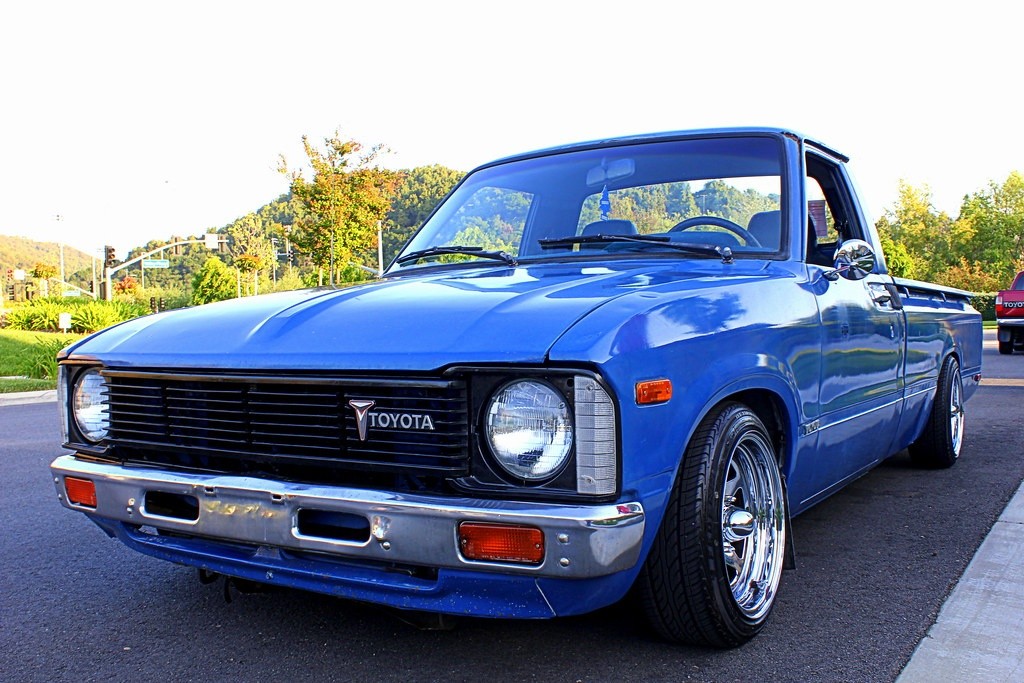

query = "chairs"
[
  {"left": 746, "top": 210, "right": 831, "bottom": 266},
  {"left": 579, "top": 219, "right": 638, "bottom": 250}
]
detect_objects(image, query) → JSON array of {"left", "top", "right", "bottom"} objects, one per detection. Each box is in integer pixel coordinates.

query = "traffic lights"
[
  {"left": 150, "top": 297, "right": 155, "bottom": 308},
  {"left": 105, "top": 245, "right": 117, "bottom": 267},
  {"left": 160, "top": 298, "right": 165, "bottom": 308},
  {"left": 89, "top": 281, "right": 93, "bottom": 292},
  {"left": 288, "top": 250, "right": 294, "bottom": 262}
]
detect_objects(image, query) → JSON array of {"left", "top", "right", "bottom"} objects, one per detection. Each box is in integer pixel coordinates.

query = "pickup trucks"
[
  {"left": 50, "top": 125, "right": 984, "bottom": 653},
  {"left": 994, "top": 271, "right": 1024, "bottom": 355}
]
